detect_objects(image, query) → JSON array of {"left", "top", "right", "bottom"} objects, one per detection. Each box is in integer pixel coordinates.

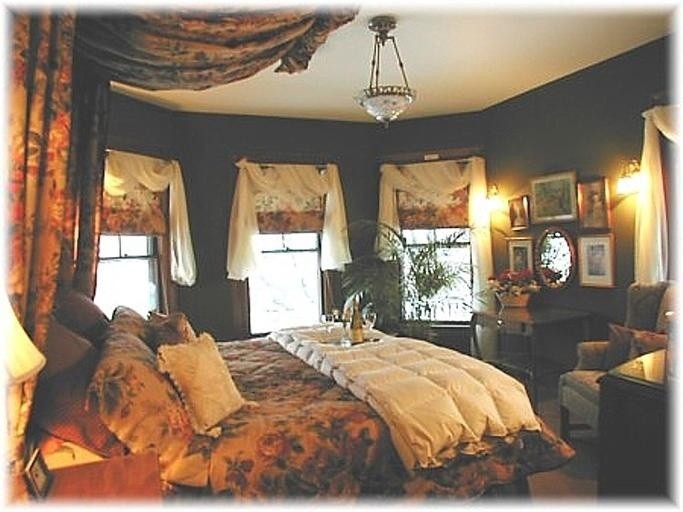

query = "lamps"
[
  {"left": 352, "top": 14, "right": 418, "bottom": 129},
  {"left": 6, "top": 289, "right": 48, "bottom": 386}
]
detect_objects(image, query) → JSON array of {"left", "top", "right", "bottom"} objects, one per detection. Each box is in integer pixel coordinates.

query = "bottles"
[{"left": 348, "top": 303, "right": 363, "bottom": 342}]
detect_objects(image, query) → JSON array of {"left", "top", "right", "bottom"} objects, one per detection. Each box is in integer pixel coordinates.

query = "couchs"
[{"left": 558, "top": 280, "right": 679, "bottom": 443}]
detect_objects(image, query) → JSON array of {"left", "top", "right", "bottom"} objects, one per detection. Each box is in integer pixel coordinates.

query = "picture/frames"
[
  {"left": 508, "top": 195, "right": 530, "bottom": 231},
  {"left": 577, "top": 232, "right": 616, "bottom": 289},
  {"left": 576, "top": 176, "right": 611, "bottom": 230},
  {"left": 529, "top": 168, "right": 579, "bottom": 225},
  {"left": 23, "top": 447, "right": 53, "bottom": 501},
  {"left": 505, "top": 236, "right": 536, "bottom": 278}
]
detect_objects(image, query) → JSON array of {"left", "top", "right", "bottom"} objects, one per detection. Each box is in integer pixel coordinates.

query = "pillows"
[
  {"left": 606, "top": 321, "right": 668, "bottom": 370},
  {"left": 30, "top": 286, "right": 245, "bottom": 488}
]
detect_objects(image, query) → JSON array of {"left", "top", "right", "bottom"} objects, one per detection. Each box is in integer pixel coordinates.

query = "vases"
[{"left": 495, "top": 291, "right": 530, "bottom": 308}]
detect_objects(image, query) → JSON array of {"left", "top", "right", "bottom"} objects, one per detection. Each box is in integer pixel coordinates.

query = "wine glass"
[
  {"left": 320, "top": 314, "right": 334, "bottom": 339},
  {"left": 362, "top": 310, "right": 378, "bottom": 339}
]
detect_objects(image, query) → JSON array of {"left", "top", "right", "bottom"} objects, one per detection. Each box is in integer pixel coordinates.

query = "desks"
[
  {"left": 596, "top": 349, "right": 671, "bottom": 498},
  {"left": 469, "top": 304, "right": 592, "bottom": 423}
]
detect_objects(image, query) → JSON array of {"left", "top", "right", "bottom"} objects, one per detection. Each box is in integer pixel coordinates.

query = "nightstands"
[{"left": 26, "top": 452, "right": 165, "bottom": 503}]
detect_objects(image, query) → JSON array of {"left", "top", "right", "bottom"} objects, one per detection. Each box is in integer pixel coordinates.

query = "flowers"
[{"left": 485, "top": 268, "right": 540, "bottom": 297}]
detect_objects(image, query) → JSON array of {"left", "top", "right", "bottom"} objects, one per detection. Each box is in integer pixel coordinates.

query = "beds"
[{"left": 22, "top": 320, "right": 578, "bottom": 503}]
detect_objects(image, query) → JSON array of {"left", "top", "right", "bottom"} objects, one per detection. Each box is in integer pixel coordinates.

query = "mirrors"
[{"left": 534, "top": 226, "right": 577, "bottom": 292}]
